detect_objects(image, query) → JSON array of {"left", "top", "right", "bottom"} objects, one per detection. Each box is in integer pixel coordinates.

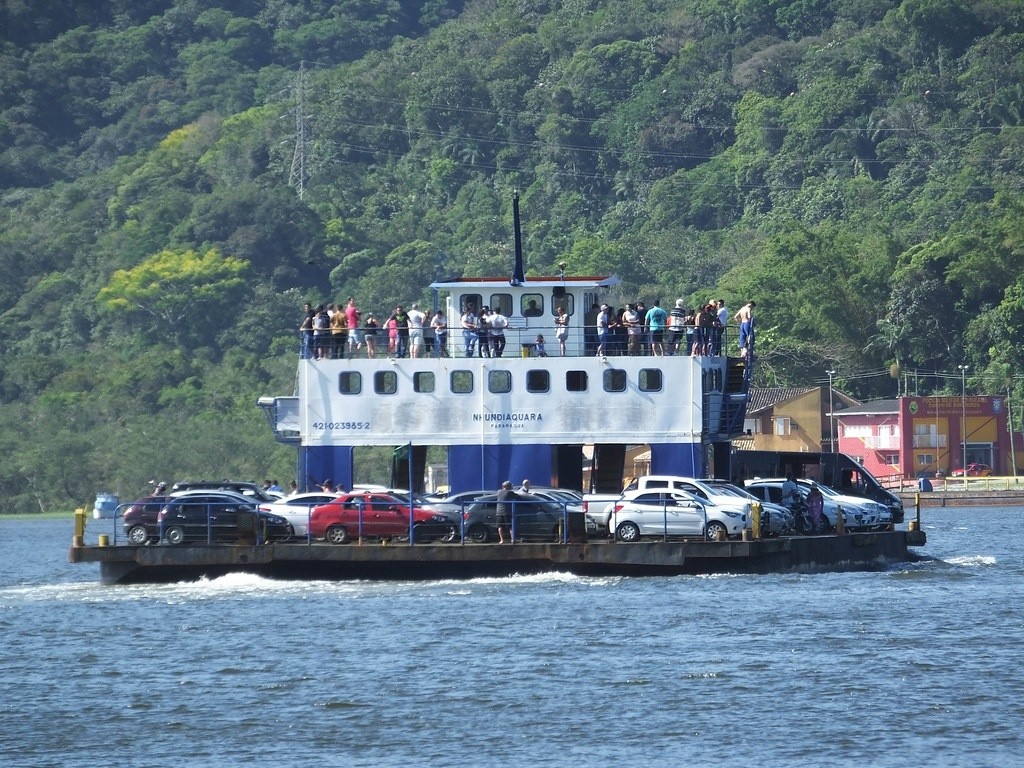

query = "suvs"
[{"left": 168, "top": 479, "right": 285, "bottom": 506}]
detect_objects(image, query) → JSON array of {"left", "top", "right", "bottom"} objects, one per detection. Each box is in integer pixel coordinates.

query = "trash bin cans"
[
  {"left": 522, "top": 344, "right": 533, "bottom": 358},
  {"left": 566, "top": 510, "right": 587, "bottom": 543}
]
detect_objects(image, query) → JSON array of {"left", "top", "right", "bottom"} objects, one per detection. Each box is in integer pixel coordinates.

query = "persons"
[
  {"left": 735, "top": 301, "right": 756, "bottom": 357},
  {"left": 460, "top": 302, "right": 508, "bottom": 357},
  {"left": 782, "top": 472, "right": 797, "bottom": 504},
  {"left": 335, "top": 483, "right": 345, "bottom": 494},
  {"left": 731, "top": 476, "right": 737, "bottom": 485},
  {"left": 148, "top": 480, "right": 167, "bottom": 496},
  {"left": 524, "top": 300, "right": 542, "bottom": 315},
  {"left": 299, "top": 297, "right": 449, "bottom": 357},
  {"left": 535, "top": 334, "right": 546, "bottom": 357},
  {"left": 807, "top": 482, "right": 824, "bottom": 535},
  {"left": 315, "top": 479, "right": 332, "bottom": 492},
  {"left": 844, "top": 471, "right": 853, "bottom": 487},
  {"left": 289, "top": 481, "right": 298, "bottom": 495},
  {"left": 585, "top": 298, "right": 727, "bottom": 356},
  {"left": 262, "top": 479, "right": 283, "bottom": 492},
  {"left": 519, "top": 480, "right": 530, "bottom": 493},
  {"left": 495, "top": 481, "right": 518, "bottom": 544},
  {"left": 555, "top": 307, "right": 569, "bottom": 357}
]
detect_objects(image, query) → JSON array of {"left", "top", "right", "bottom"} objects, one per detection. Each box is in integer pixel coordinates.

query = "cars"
[
  {"left": 256, "top": 488, "right": 364, "bottom": 538},
  {"left": 609, "top": 488, "right": 748, "bottom": 542},
  {"left": 156, "top": 491, "right": 289, "bottom": 546},
  {"left": 121, "top": 496, "right": 182, "bottom": 546},
  {"left": 305, "top": 491, "right": 449, "bottom": 543},
  {"left": 461, "top": 492, "right": 565, "bottom": 544},
  {"left": 951, "top": 463, "right": 994, "bottom": 478},
  {"left": 351, "top": 477, "right": 893, "bottom": 537}
]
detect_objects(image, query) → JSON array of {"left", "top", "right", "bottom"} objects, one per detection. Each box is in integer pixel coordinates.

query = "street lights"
[
  {"left": 959, "top": 364, "right": 971, "bottom": 491},
  {"left": 824, "top": 368, "right": 838, "bottom": 454}
]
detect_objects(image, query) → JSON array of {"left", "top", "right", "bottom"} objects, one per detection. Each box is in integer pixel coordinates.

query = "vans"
[{"left": 731, "top": 447, "right": 906, "bottom": 524}]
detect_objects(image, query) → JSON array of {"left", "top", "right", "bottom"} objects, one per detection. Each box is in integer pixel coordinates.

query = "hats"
[
  {"left": 809, "top": 482, "right": 818, "bottom": 489},
  {"left": 600, "top": 304, "right": 608, "bottom": 311},
  {"left": 335, "top": 484, "right": 345, "bottom": 488}
]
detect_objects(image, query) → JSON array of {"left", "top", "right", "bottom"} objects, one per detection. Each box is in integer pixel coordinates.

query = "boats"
[
  {"left": 92, "top": 492, "right": 122, "bottom": 519},
  {"left": 68, "top": 190, "right": 930, "bottom": 584}
]
